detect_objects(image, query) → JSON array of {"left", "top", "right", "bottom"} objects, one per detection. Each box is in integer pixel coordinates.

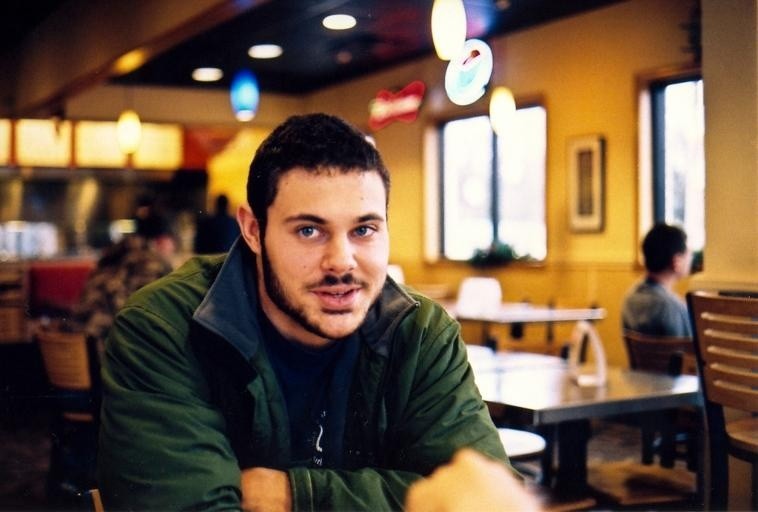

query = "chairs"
[
  {"left": 31, "top": 328, "right": 104, "bottom": 509},
  {"left": 493, "top": 289, "right": 601, "bottom": 364},
  {"left": 684, "top": 289, "right": 758, "bottom": 511}
]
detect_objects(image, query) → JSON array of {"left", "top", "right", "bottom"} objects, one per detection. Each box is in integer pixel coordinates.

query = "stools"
[{"left": 489, "top": 426, "right": 546, "bottom": 460}]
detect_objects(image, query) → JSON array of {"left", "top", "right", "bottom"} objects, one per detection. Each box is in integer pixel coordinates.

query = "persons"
[
  {"left": 621, "top": 221, "right": 710, "bottom": 494},
  {"left": 97, "top": 193, "right": 241, "bottom": 268},
  {"left": 97, "top": 110, "right": 544, "bottom": 511}
]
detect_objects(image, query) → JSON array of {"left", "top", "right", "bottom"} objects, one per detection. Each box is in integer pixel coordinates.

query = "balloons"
[{"left": 465, "top": 2, "right": 495, "bottom": 40}]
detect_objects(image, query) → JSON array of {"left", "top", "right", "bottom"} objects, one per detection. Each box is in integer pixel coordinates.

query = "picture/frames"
[{"left": 564, "top": 134, "right": 605, "bottom": 233}]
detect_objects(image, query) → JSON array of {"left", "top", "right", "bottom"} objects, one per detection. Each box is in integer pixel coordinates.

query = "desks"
[
  {"left": 425, "top": 301, "right": 605, "bottom": 352},
  {"left": 458, "top": 344, "right": 702, "bottom": 510}
]
